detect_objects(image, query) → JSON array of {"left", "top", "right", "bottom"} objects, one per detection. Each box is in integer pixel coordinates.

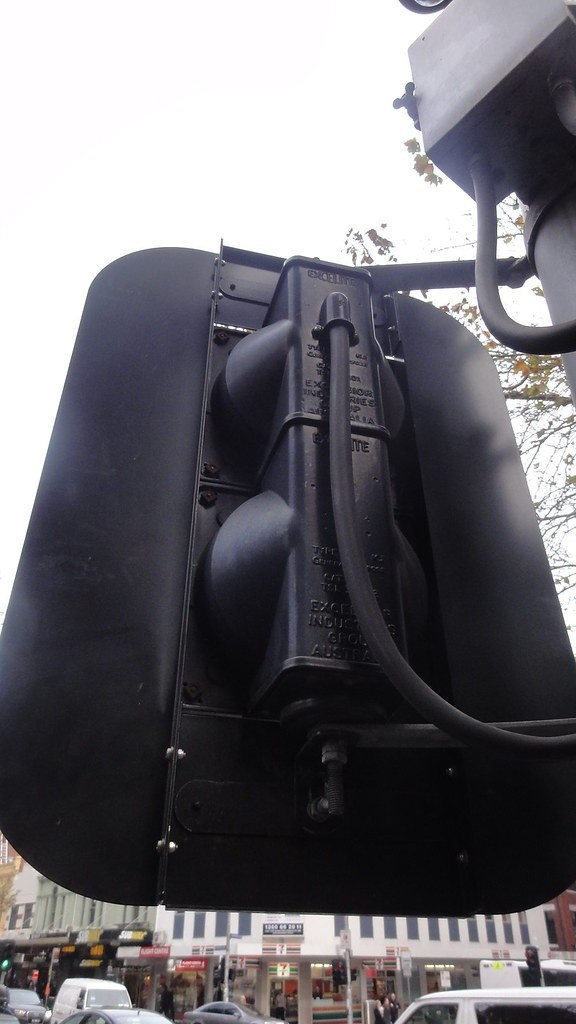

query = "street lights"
[
  {"left": 525, "top": 945, "right": 543, "bottom": 980},
  {"left": 224, "top": 932, "right": 243, "bottom": 1001}
]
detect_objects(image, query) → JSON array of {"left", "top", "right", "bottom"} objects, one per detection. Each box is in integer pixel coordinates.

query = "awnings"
[{"left": 26, "top": 928, "right": 151, "bottom": 947}]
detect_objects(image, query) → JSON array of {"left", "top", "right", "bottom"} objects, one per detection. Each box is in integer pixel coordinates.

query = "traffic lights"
[
  {"left": 331, "top": 958, "right": 347, "bottom": 985},
  {"left": 0, "top": 938, "right": 16, "bottom": 972},
  {"left": 351, "top": 969, "right": 357, "bottom": 981}
]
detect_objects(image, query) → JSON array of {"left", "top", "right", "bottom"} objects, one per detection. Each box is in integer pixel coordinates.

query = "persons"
[
  {"left": 275, "top": 989, "right": 287, "bottom": 1020},
  {"left": 312, "top": 986, "right": 322, "bottom": 999},
  {"left": 374, "top": 992, "right": 401, "bottom": 1024},
  {"left": 198, "top": 985, "right": 204, "bottom": 1009},
  {"left": 10, "top": 974, "right": 57, "bottom": 1001},
  {"left": 158, "top": 983, "right": 175, "bottom": 1022},
  {"left": 213, "top": 984, "right": 223, "bottom": 1002}
]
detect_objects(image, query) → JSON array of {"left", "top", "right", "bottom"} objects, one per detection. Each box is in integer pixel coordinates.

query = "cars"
[
  {"left": 8, "top": 987, "right": 47, "bottom": 1024},
  {"left": 182, "top": 1001, "right": 289, "bottom": 1024},
  {"left": 57, "top": 1005, "right": 174, "bottom": 1024},
  {"left": 392, "top": 985, "right": 576, "bottom": 1024}
]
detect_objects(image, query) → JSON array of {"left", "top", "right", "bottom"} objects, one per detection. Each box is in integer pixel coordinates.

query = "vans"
[
  {"left": 50, "top": 978, "right": 132, "bottom": 1024},
  {"left": 480, "top": 960, "right": 575, "bottom": 987}
]
